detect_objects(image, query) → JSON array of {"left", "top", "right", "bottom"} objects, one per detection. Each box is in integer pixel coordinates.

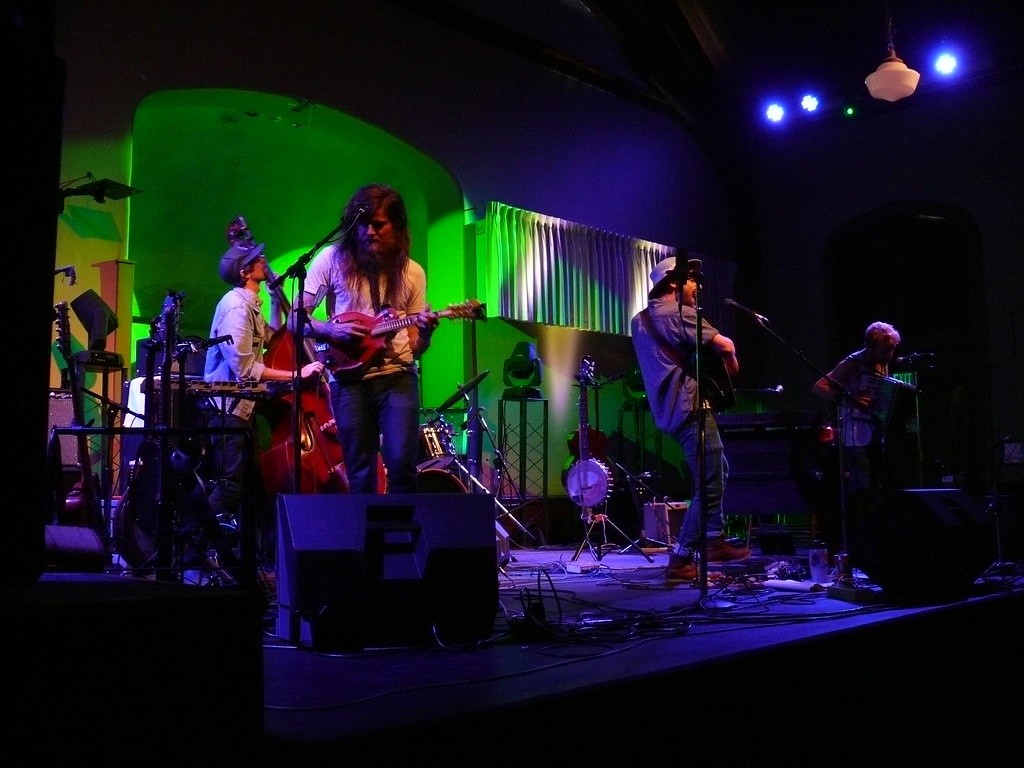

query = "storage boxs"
[{"left": 643, "top": 501, "right": 689, "bottom": 543}]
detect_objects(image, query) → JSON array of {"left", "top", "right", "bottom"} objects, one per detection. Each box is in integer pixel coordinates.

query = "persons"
[
  {"left": 201, "top": 244, "right": 324, "bottom": 516},
  {"left": 631, "top": 257, "right": 751, "bottom": 583},
  {"left": 286, "top": 186, "right": 439, "bottom": 496},
  {"left": 813, "top": 321, "right": 902, "bottom": 576}
]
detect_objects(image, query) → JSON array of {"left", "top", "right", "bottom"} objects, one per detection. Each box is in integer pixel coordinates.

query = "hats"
[
  {"left": 220, "top": 242, "right": 265, "bottom": 284},
  {"left": 647, "top": 257, "right": 703, "bottom": 299}
]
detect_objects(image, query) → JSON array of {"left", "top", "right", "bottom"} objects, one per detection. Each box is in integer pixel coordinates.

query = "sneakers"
[
  {"left": 665, "top": 551, "right": 721, "bottom": 582},
  {"left": 705, "top": 535, "right": 752, "bottom": 565}
]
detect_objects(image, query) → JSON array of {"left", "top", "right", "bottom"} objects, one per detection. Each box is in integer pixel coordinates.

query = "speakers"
[
  {"left": 643, "top": 502, "right": 690, "bottom": 548},
  {"left": 42, "top": 525, "right": 109, "bottom": 574},
  {"left": 852, "top": 487, "right": 997, "bottom": 605},
  {"left": 276, "top": 492, "right": 499, "bottom": 651}
]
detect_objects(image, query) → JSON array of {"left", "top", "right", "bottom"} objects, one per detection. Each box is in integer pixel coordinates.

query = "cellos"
[{"left": 226, "top": 215, "right": 388, "bottom": 523}]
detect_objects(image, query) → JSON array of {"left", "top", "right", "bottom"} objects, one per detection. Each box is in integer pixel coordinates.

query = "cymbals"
[{"left": 436, "top": 368, "right": 489, "bottom": 414}]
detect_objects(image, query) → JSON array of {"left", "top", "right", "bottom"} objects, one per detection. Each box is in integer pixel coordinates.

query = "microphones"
[
  {"left": 461, "top": 408, "right": 481, "bottom": 430},
  {"left": 357, "top": 201, "right": 369, "bottom": 216},
  {"left": 897, "top": 353, "right": 929, "bottom": 363},
  {"left": 724, "top": 298, "right": 770, "bottom": 323},
  {"left": 675, "top": 247, "right": 688, "bottom": 306}
]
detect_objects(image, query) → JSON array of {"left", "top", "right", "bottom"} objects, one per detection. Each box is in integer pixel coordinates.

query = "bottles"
[{"left": 809, "top": 540, "right": 828, "bottom": 582}]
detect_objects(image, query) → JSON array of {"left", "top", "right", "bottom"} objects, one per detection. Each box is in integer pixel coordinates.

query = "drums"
[{"left": 397, "top": 421, "right": 470, "bottom": 493}]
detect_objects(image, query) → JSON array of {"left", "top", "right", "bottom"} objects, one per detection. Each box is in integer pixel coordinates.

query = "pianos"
[{"left": 140, "top": 378, "right": 273, "bottom": 399}]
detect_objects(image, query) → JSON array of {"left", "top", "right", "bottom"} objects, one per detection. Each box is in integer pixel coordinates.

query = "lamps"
[
  {"left": 65, "top": 280, "right": 126, "bottom": 368},
  {"left": 864, "top": 0, "right": 921, "bottom": 101}
]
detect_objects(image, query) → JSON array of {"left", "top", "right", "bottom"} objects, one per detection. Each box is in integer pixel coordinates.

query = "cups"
[{"left": 834, "top": 554, "right": 853, "bottom": 579}]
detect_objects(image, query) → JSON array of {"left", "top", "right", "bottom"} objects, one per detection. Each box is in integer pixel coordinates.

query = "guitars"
[
  {"left": 565, "top": 354, "right": 614, "bottom": 507},
  {"left": 699, "top": 312, "right": 738, "bottom": 412},
  {"left": 54, "top": 298, "right": 109, "bottom": 557},
  {"left": 112, "top": 291, "right": 216, "bottom": 574},
  {"left": 319, "top": 298, "right": 484, "bottom": 372}
]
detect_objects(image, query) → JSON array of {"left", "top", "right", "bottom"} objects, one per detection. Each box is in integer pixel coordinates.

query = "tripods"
[{"left": 571, "top": 382, "right": 674, "bottom": 564}]
